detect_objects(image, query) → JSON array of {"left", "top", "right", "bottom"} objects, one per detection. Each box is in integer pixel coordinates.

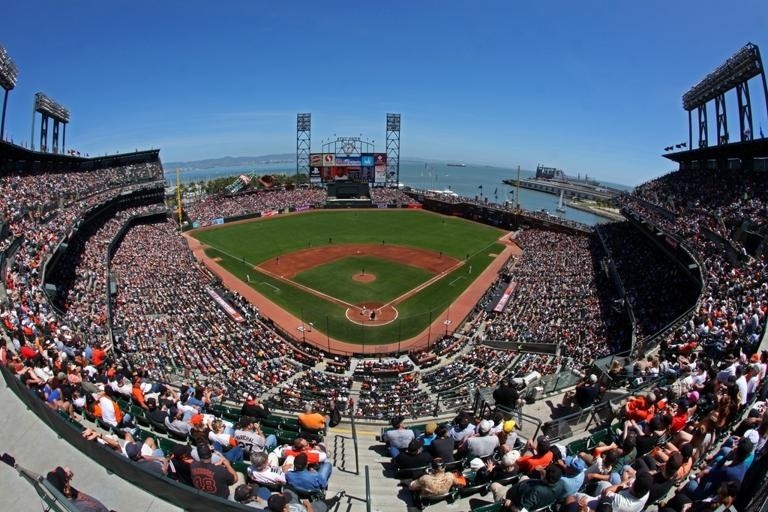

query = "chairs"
[{"left": 77, "top": 396, "right": 328, "bottom": 512}]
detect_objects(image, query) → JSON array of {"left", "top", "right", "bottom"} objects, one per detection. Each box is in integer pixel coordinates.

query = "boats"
[{"left": 448, "top": 163, "right": 467, "bottom": 167}]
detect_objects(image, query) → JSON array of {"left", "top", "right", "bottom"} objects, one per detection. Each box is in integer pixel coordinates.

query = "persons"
[
  {"left": 1, "top": 163, "right": 239, "bottom": 512},
  {"left": 488, "top": 166, "right": 767, "bottom": 512},
  {"left": 375, "top": 170, "right": 386, "bottom": 183},
  {"left": 335, "top": 166, "right": 348, "bottom": 180},
  {"left": 324, "top": 170, "right": 334, "bottom": 182},
  {"left": 239, "top": 174, "right": 488, "bottom": 214},
  {"left": 236, "top": 290, "right": 489, "bottom": 512}
]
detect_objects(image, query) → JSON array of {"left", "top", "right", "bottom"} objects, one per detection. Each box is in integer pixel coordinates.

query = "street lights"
[
  {"left": 296, "top": 112, "right": 312, "bottom": 180},
  {"left": 385, "top": 112, "right": 402, "bottom": 187}
]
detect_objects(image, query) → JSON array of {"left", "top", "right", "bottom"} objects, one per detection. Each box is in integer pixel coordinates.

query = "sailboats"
[{"left": 556, "top": 188, "right": 567, "bottom": 212}]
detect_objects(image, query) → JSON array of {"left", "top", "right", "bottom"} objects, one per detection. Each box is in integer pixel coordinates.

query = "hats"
[
  {"left": 502, "top": 450, "right": 520, "bottom": 466},
  {"left": 140, "top": 383, "right": 152, "bottom": 395},
  {"left": 546, "top": 456, "right": 585, "bottom": 482},
  {"left": 668, "top": 451, "right": 682, "bottom": 472},
  {"left": 538, "top": 436, "right": 549, "bottom": 445},
  {"left": 687, "top": 391, "right": 700, "bottom": 401},
  {"left": 635, "top": 471, "right": 653, "bottom": 492},
  {"left": 391, "top": 416, "right": 437, "bottom": 451},
  {"left": 590, "top": 374, "right": 598, "bottom": 383},
  {"left": 329, "top": 409, "right": 341, "bottom": 426},
  {"left": 126, "top": 441, "right": 142, "bottom": 458},
  {"left": 47, "top": 467, "right": 73, "bottom": 488},
  {"left": 235, "top": 482, "right": 292, "bottom": 509},
  {"left": 240, "top": 416, "right": 256, "bottom": 427},
  {"left": 175, "top": 443, "right": 211, "bottom": 459},
  {"left": 295, "top": 453, "right": 307, "bottom": 468},
  {"left": 191, "top": 415, "right": 203, "bottom": 424},
  {"left": 480, "top": 419, "right": 515, "bottom": 432},
  {"left": 744, "top": 429, "right": 759, "bottom": 443}
]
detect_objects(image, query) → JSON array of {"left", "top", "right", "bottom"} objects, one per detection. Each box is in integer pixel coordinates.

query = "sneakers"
[{"left": 335, "top": 491, "right": 345, "bottom": 500}]
